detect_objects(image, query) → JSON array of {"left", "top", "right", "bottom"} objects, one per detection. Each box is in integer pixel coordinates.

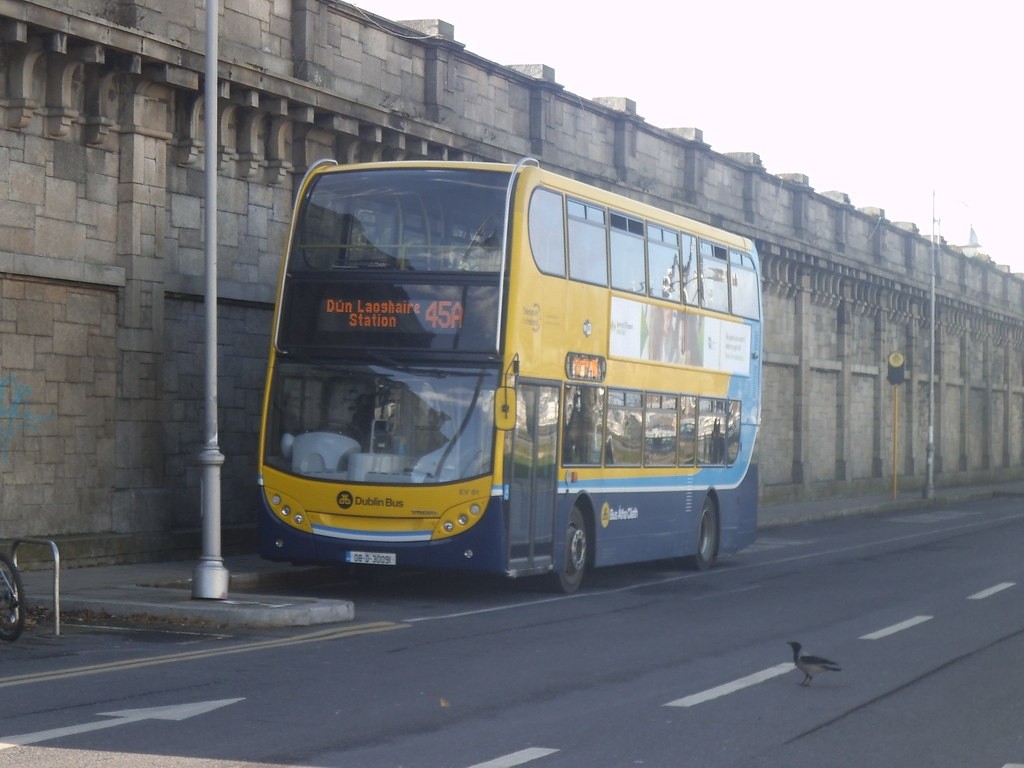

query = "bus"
[{"left": 256, "top": 154, "right": 764, "bottom": 597}]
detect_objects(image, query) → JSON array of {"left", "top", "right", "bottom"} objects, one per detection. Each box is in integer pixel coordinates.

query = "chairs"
[{"left": 352, "top": 394, "right": 388, "bottom": 434}]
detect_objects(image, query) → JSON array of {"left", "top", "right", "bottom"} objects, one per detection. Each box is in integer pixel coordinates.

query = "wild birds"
[{"left": 784, "top": 640, "right": 843, "bottom": 688}]
